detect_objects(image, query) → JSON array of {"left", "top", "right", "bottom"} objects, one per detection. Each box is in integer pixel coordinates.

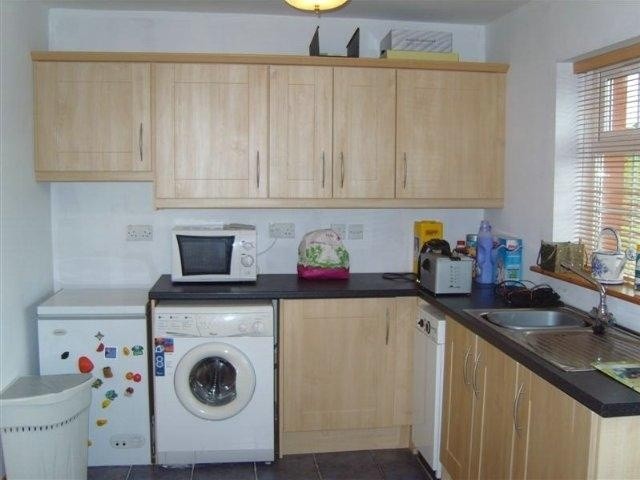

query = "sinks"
[{"left": 460, "top": 308, "right": 594, "bottom": 330}]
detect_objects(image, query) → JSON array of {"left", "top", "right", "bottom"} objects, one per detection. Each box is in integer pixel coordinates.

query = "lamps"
[{"left": 284, "top": 0, "right": 351, "bottom": 16}]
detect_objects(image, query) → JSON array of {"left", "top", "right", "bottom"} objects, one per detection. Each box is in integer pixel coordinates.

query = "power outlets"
[
  {"left": 269, "top": 224, "right": 295, "bottom": 238},
  {"left": 331, "top": 224, "right": 346, "bottom": 239},
  {"left": 348, "top": 225, "right": 363, "bottom": 240},
  {"left": 125, "top": 225, "right": 153, "bottom": 241}
]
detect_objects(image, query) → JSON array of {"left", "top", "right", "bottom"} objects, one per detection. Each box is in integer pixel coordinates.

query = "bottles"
[{"left": 466, "top": 234, "right": 478, "bottom": 279}]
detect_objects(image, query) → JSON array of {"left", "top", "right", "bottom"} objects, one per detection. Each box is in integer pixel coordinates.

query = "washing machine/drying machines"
[{"left": 153, "top": 301, "right": 274, "bottom": 467}]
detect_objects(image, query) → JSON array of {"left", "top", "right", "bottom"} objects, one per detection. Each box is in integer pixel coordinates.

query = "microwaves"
[{"left": 170, "top": 223, "right": 259, "bottom": 283}]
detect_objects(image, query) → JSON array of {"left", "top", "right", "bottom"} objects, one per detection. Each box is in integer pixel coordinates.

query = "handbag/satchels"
[{"left": 298, "top": 230, "right": 349, "bottom": 280}]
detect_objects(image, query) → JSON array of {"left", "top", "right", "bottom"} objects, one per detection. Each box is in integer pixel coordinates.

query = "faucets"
[{"left": 560, "top": 260, "right": 606, "bottom": 313}]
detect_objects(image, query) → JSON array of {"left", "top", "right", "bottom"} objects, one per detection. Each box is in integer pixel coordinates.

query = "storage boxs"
[{"left": 380, "top": 28, "right": 452, "bottom": 55}]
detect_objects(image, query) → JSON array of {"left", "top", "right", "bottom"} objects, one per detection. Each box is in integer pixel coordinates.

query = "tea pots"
[{"left": 590, "top": 227, "right": 633, "bottom": 285}]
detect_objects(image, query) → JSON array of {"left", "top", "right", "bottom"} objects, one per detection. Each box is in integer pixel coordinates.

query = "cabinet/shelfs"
[
  {"left": 438, "top": 309, "right": 519, "bottom": 476},
  {"left": 280, "top": 297, "right": 418, "bottom": 454},
  {"left": 152, "top": 49, "right": 269, "bottom": 208},
  {"left": 34, "top": 52, "right": 152, "bottom": 179},
  {"left": 527, "top": 352, "right": 640, "bottom": 479},
  {"left": 268, "top": 52, "right": 396, "bottom": 208},
  {"left": 394, "top": 59, "right": 506, "bottom": 208}
]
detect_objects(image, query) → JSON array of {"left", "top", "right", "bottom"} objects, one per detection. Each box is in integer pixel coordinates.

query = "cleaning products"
[{"left": 475, "top": 220, "right": 493, "bottom": 284}]
